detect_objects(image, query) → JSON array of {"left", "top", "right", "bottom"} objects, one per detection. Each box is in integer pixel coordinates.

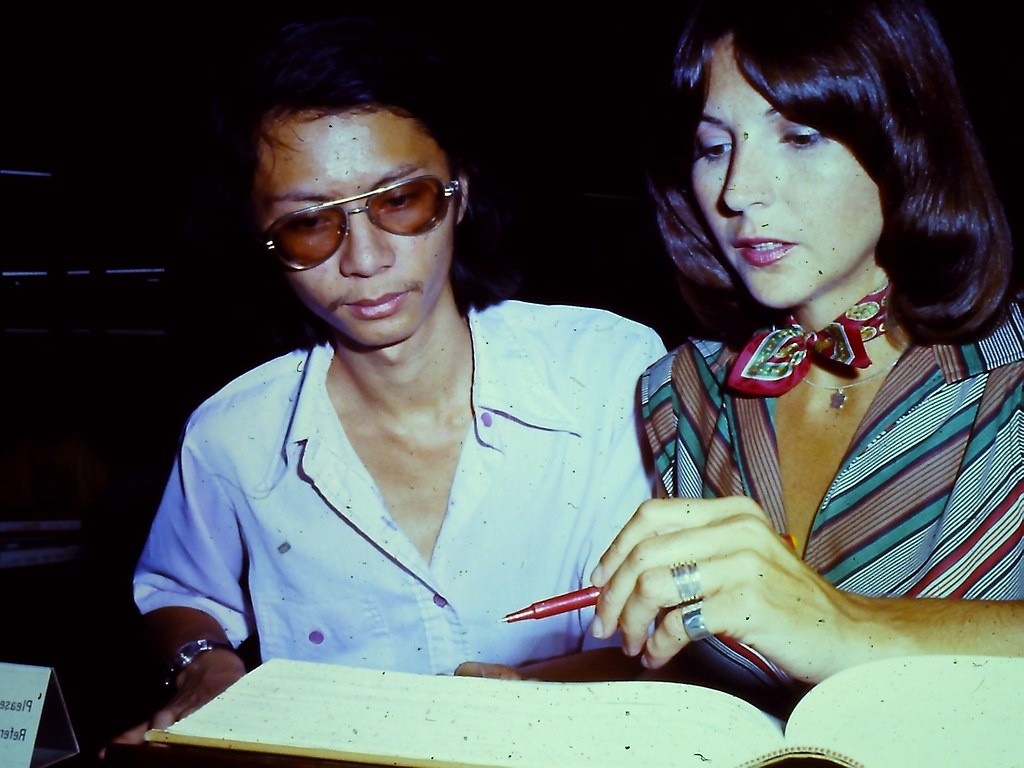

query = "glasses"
[{"left": 257, "top": 177, "right": 461, "bottom": 270}]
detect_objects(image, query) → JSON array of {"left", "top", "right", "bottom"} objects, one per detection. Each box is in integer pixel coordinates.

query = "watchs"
[{"left": 164, "top": 638, "right": 236, "bottom": 687}]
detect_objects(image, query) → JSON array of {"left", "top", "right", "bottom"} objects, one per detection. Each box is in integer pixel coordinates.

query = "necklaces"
[{"left": 801, "top": 361, "right": 895, "bottom": 408}]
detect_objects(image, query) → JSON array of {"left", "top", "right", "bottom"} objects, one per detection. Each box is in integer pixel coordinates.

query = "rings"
[
  {"left": 682, "top": 603, "right": 711, "bottom": 644},
  {"left": 671, "top": 559, "right": 701, "bottom": 601}
]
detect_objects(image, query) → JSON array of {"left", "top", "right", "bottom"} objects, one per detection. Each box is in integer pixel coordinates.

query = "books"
[{"left": 148, "top": 652, "right": 1024, "bottom": 768}]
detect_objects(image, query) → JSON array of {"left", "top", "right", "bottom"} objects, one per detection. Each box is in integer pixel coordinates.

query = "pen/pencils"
[{"left": 500, "top": 586, "right": 604, "bottom": 623}]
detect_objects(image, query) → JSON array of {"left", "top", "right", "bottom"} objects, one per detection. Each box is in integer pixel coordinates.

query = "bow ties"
[{"left": 727, "top": 280, "right": 898, "bottom": 397}]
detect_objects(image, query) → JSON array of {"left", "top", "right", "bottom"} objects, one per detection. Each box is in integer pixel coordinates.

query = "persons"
[
  {"left": 99, "top": 16, "right": 673, "bottom": 757},
  {"left": 590, "top": 0, "right": 1024, "bottom": 714}
]
